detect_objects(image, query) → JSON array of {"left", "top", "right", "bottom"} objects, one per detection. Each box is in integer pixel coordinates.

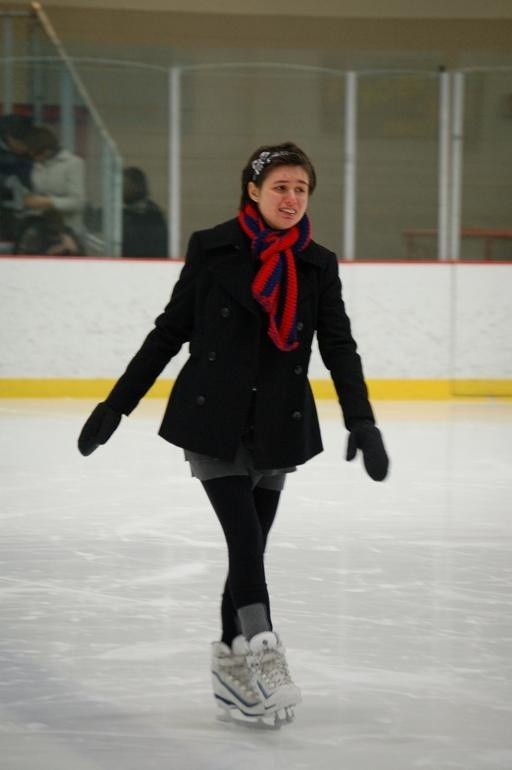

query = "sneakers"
[
  {"left": 212, "top": 636, "right": 264, "bottom": 716},
  {"left": 244, "top": 630, "right": 301, "bottom": 713}
]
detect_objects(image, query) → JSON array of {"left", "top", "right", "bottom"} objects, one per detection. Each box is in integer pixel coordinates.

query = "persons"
[
  {"left": 1, "top": 116, "right": 87, "bottom": 257},
  {"left": 79, "top": 142, "right": 388, "bottom": 716},
  {"left": 85, "top": 166, "right": 167, "bottom": 259}
]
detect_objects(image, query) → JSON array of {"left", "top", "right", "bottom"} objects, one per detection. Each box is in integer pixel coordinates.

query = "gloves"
[
  {"left": 346, "top": 425, "right": 389, "bottom": 481},
  {"left": 77, "top": 401, "right": 120, "bottom": 457}
]
